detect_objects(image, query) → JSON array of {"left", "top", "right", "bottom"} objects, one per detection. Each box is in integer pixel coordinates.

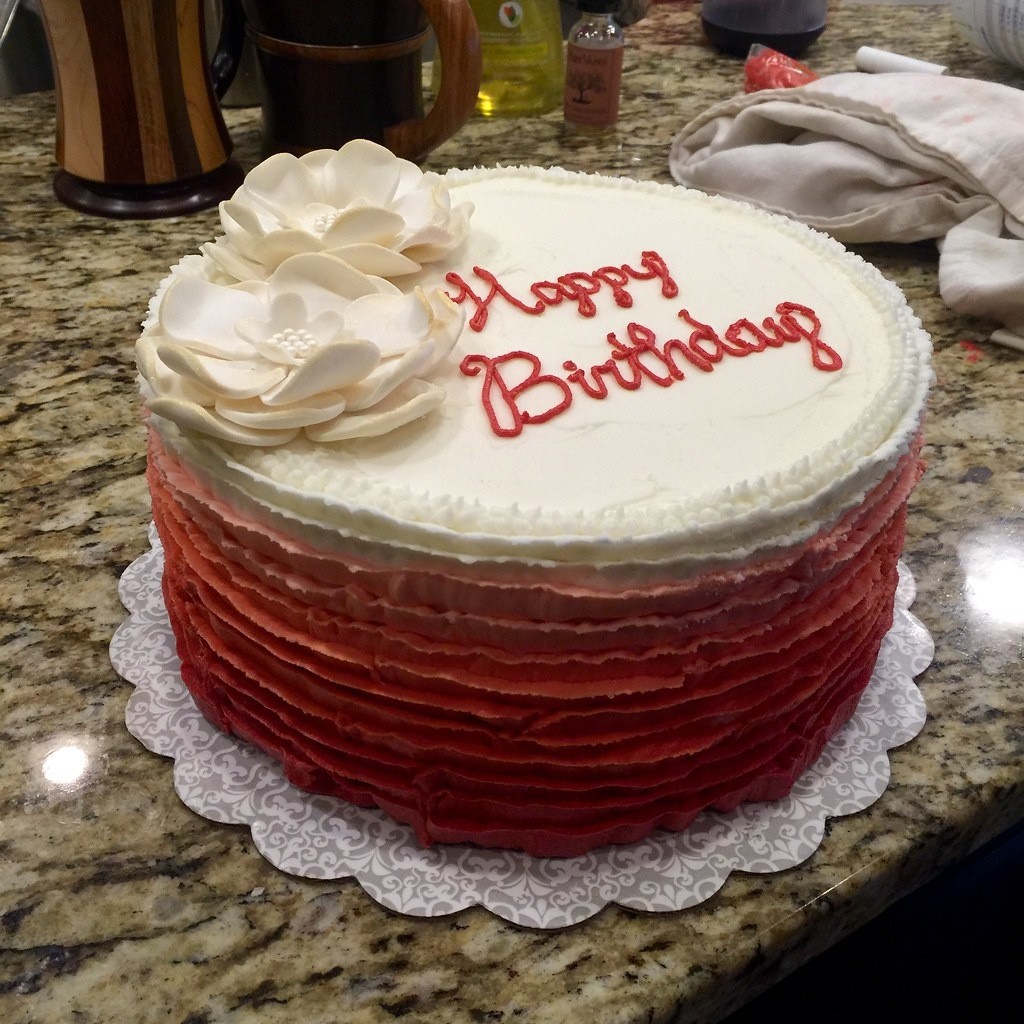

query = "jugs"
[
  {"left": 430, "top": 0, "right": 562, "bottom": 117},
  {"left": 36, "top": 0, "right": 243, "bottom": 217},
  {"left": 250, "top": 0, "right": 481, "bottom": 169}
]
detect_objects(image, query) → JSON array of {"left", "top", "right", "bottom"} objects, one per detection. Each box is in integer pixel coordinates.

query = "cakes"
[{"left": 132, "top": 137, "right": 938, "bottom": 861}]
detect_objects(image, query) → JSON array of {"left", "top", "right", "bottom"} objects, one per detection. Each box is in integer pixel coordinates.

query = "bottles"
[{"left": 562, "top": 0, "right": 624, "bottom": 134}]
